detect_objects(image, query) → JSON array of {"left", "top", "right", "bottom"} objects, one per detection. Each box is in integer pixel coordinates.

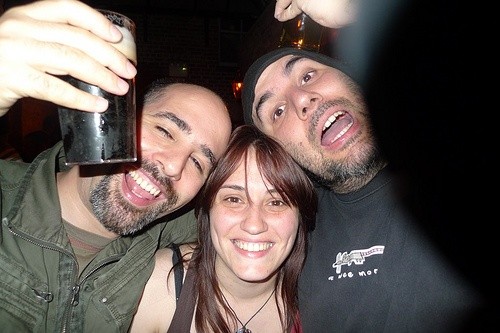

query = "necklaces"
[{"left": 214, "top": 279, "right": 279, "bottom": 333}]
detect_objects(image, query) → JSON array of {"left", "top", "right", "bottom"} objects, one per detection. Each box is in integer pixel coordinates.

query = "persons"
[
  {"left": 0, "top": 0, "right": 232, "bottom": 333},
  {"left": 241, "top": 0, "right": 500, "bottom": 333},
  {"left": 129, "top": 124, "right": 317, "bottom": 333}
]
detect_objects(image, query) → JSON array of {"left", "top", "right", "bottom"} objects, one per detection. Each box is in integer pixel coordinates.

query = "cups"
[
  {"left": 57, "top": 8, "right": 137, "bottom": 165},
  {"left": 278, "top": 11, "right": 323, "bottom": 54}
]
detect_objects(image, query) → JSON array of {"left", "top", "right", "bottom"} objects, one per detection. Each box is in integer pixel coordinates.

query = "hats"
[{"left": 243, "top": 47, "right": 348, "bottom": 123}]
